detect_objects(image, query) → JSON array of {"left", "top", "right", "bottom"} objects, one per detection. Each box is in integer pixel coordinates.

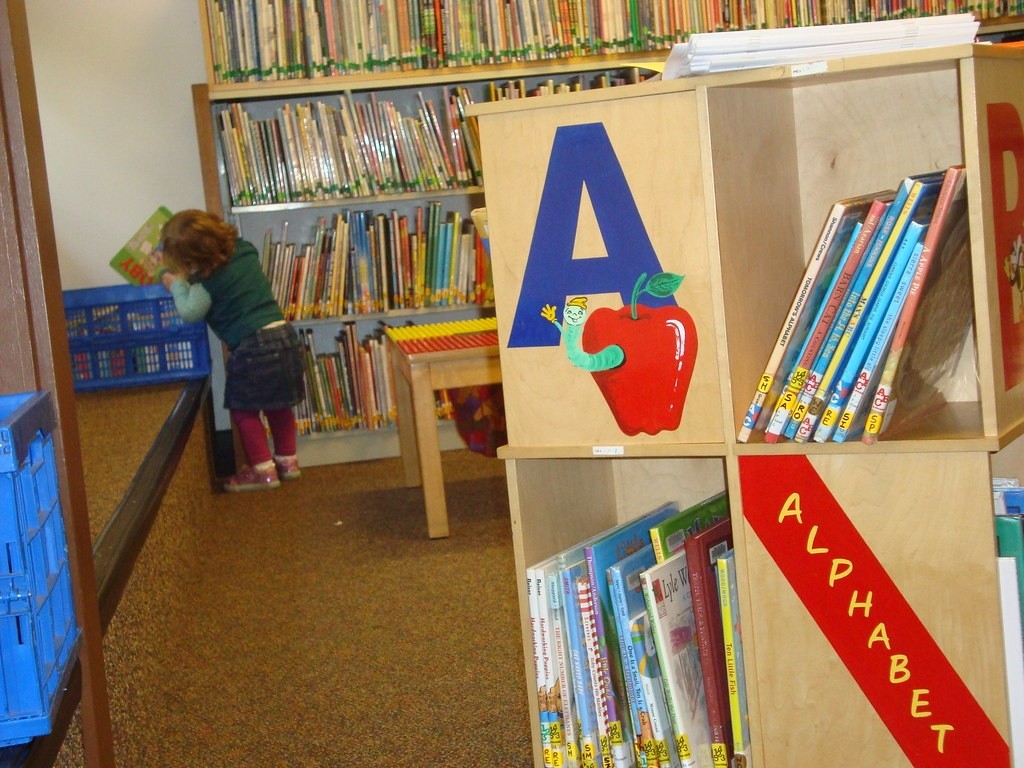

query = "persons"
[{"left": 151, "top": 210, "right": 308, "bottom": 491}]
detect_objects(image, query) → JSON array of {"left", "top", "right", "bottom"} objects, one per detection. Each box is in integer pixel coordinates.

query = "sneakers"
[
  {"left": 224, "top": 464, "right": 280, "bottom": 489},
  {"left": 272, "top": 455, "right": 300, "bottom": 480}
]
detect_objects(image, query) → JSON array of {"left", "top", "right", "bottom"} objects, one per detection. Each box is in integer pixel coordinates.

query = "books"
[
  {"left": 205, "top": 1, "right": 1024, "bottom": 444},
  {"left": 522, "top": 478, "right": 1023, "bottom": 768},
  {"left": 110, "top": 206, "right": 190, "bottom": 289},
  {"left": 62, "top": 303, "right": 193, "bottom": 381}
]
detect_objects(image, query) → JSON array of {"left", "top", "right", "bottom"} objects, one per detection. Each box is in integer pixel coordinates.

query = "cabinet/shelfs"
[{"left": 191, "top": 14, "right": 1024, "bottom": 768}]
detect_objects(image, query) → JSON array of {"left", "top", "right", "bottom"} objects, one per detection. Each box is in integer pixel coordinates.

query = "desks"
[{"left": 383, "top": 315, "right": 505, "bottom": 539}]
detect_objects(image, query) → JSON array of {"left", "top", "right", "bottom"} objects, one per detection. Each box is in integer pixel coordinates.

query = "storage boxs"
[
  {"left": 0, "top": 390, "right": 80, "bottom": 752},
  {"left": 62, "top": 283, "right": 212, "bottom": 394}
]
